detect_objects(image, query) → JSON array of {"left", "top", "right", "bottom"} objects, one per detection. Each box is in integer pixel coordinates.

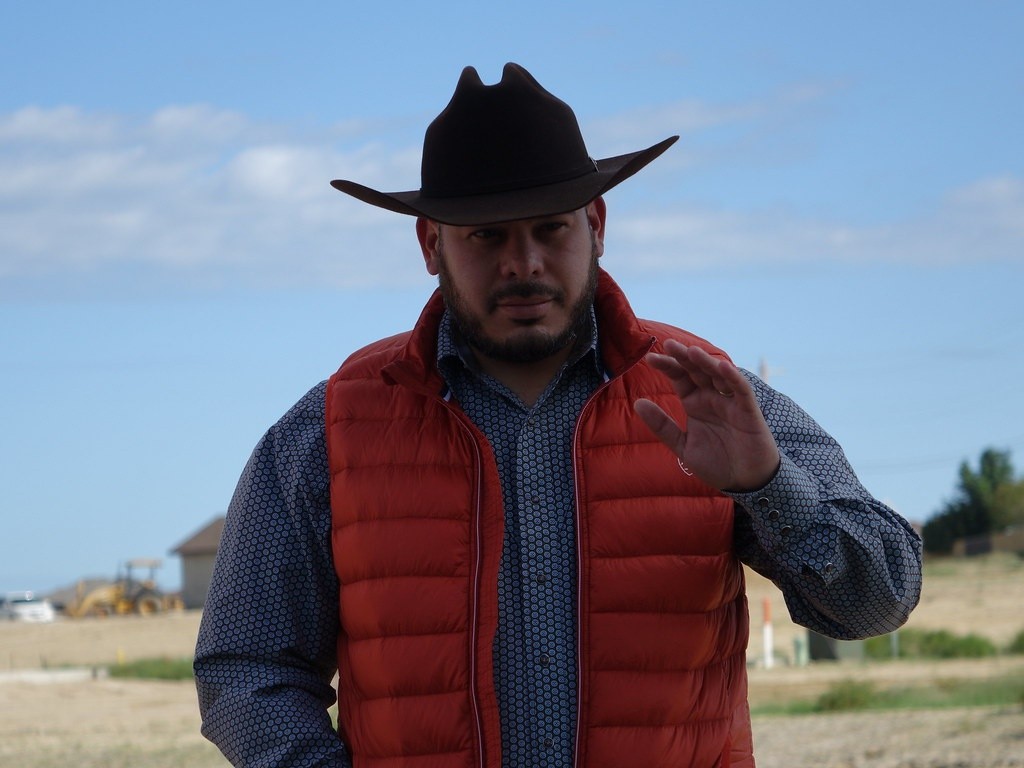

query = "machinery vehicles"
[{"left": 68, "top": 559, "right": 183, "bottom": 616}]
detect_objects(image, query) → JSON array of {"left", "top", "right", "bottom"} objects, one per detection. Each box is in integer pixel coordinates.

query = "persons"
[{"left": 193, "top": 63, "right": 922, "bottom": 768}]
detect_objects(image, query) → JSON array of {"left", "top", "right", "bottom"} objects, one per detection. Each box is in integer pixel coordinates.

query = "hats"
[{"left": 329, "top": 63, "right": 679, "bottom": 227}]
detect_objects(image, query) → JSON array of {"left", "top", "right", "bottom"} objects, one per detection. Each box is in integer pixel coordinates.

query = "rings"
[{"left": 719, "top": 392, "right": 734, "bottom": 398}]
